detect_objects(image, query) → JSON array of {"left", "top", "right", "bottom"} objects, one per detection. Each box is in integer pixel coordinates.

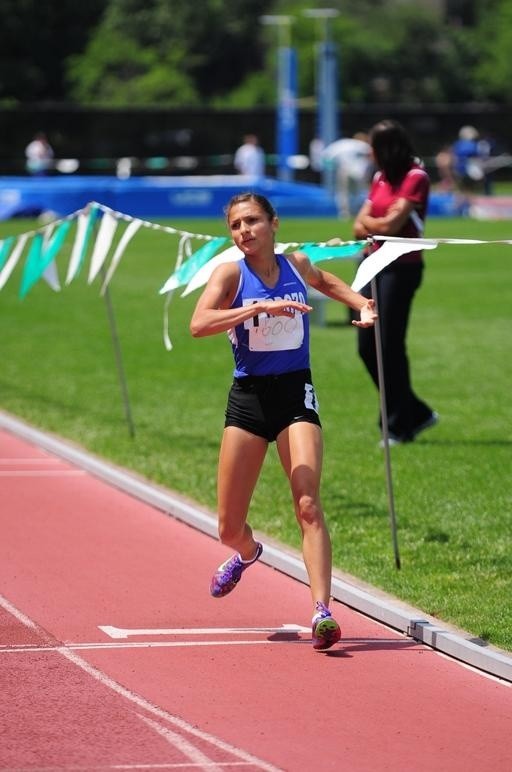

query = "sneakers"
[
  {"left": 311, "top": 614, "right": 341, "bottom": 650},
  {"left": 209, "top": 540, "right": 263, "bottom": 599},
  {"left": 376, "top": 437, "right": 416, "bottom": 449},
  {"left": 414, "top": 411, "right": 438, "bottom": 436}
]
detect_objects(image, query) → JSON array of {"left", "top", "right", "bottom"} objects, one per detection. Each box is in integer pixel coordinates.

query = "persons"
[
  {"left": 188, "top": 190, "right": 382, "bottom": 651},
  {"left": 23, "top": 131, "right": 56, "bottom": 180},
  {"left": 434, "top": 125, "right": 497, "bottom": 211},
  {"left": 348, "top": 120, "right": 439, "bottom": 451},
  {"left": 306, "top": 138, "right": 374, "bottom": 223},
  {"left": 235, "top": 132, "right": 266, "bottom": 177}
]
